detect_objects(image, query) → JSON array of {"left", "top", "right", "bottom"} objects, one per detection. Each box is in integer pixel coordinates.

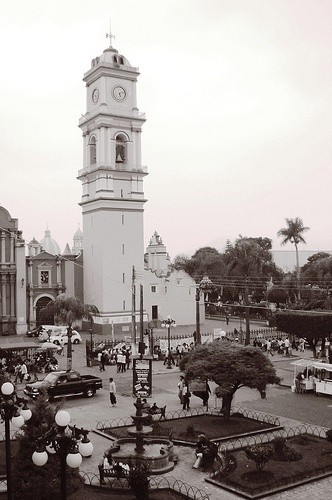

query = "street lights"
[
  {"left": 220, "top": 329, "right": 226, "bottom": 340},
  {"left": 0, "top": 381, "right": 32, "bottom": 500},
  {"left": 161, "top": 314, "right": 177, "bottom": 365},
  {"left": 33, "top": 409, "right": 94, "bottom": 500}
]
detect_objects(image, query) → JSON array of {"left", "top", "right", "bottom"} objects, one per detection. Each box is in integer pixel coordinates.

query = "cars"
[{"left": 25, "top": 325, "right": 82, "bottom": 345}]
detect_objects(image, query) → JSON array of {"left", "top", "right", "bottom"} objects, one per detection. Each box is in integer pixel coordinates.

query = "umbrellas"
[
  {"left": 35, "top": 342, "right": 63, "bottom": 357},
  {"left": 94, "top": 343, "right": 106, "bottom": 353}
]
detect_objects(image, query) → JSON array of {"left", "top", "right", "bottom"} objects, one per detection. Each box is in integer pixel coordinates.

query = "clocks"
[
  {"left": 111, "top": 83, "right": 128, "bottom": 103},
  {"left": 92, "top": 88, "right": 99, "bottom": 104}
]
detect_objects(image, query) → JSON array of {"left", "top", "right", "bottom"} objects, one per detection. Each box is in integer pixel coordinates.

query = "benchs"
[{"left": 98, "top": 464, "right": 130, "bottom": 486}]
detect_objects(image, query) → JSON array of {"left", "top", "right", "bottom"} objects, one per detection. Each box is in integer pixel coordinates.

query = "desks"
[
  {"left": 299, "top": 379, "right": 315, "bottom": 394},
  {"left": 315, "top": 381, "right": 332, "bottom": 397}
]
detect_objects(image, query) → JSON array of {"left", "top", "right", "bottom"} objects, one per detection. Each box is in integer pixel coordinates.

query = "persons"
[
  {"left": 163, "top": 340, "right": 205, "bottom": 366},
  {"left": 293, "top": 366, "right": 332, "bottom": 394},
  {"left": 142, "top": 398, "right": 150, "bottom": 410},
  {"left": 192, "top": 439, "right": 218, "bottom": 470},
  {"left": 203, "top": 296, "right": 286, "bottom": 323},
  {"left": 246, "top": 334, "right": 332, "bottom": 361},
  {"left": 152, "top": 403, "right": 160, "bottom": 411},
  {"left": 195, "top": 434, "right": 212, "bottom": 457},
  {"left": 0, "top": 348, "right": 59, "bottom": 387},
  {"left": 98, "top": 346, "right": 131, "bottom": 374},
  {"left": 109, "top": 378, "right": 117, "bottom": 407},
  {"left": 181, "top": 382, "right": 191, "bottom": 410},
  {"left": 178, "top": 376, "right": 186, "bottom": 403},
  {"left": 202, "top": 378, "right": 211, "bottom": 406}
]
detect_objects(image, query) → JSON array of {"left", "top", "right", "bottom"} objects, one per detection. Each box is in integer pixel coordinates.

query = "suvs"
[{"left": 22, "top": 370, "right": 103, "bottom": 404}]
